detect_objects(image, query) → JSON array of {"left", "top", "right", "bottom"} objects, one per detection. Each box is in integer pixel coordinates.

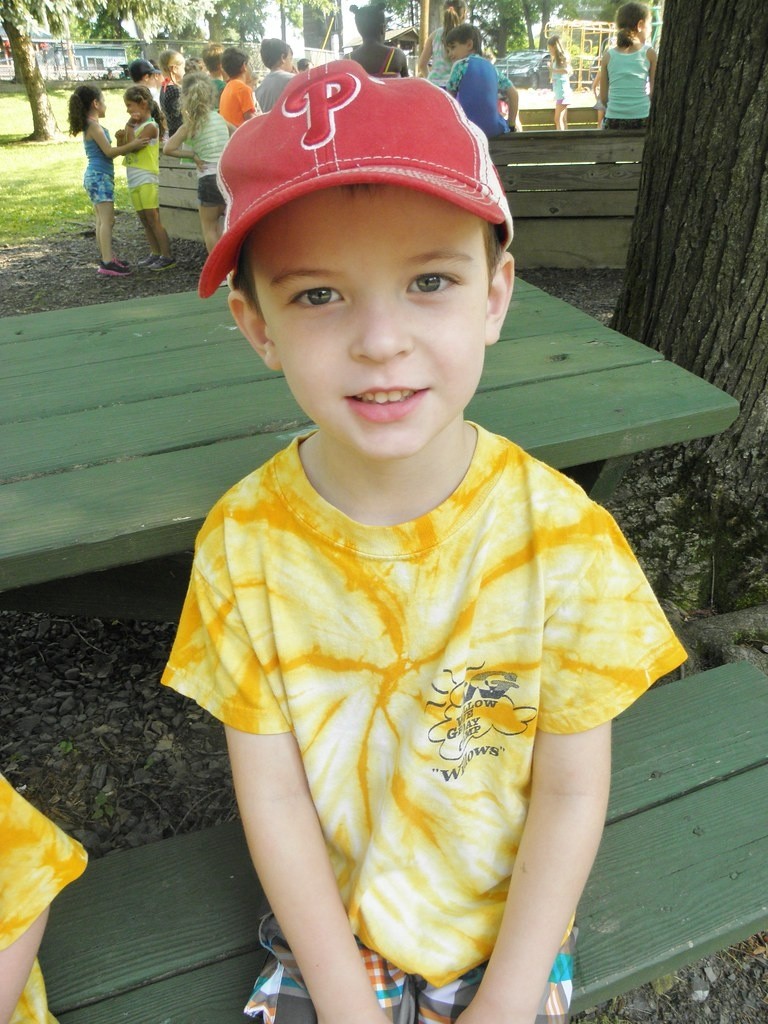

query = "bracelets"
[{"left": 126, "top": 122, "right": 136, "bottom": 128}]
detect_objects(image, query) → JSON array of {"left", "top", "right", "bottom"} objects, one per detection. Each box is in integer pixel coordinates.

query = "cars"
[{"left": 494, "top": 48, "right": 554, "bottom": 89}]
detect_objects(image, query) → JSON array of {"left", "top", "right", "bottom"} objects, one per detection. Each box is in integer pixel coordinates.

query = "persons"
[
  {"left": 444, "top": 22, "right": 519, "bottom": 135},
  {"left": 546, "top": 34, "right": 574, "bottom": 130},
  {"left": 159, "top": 57, "right": 692, "bottom": 1024},
  {"left": 66, "top": 0, "right": 522, "bottom": 277},
  {"left": 0, "top": 770, "right": 89, "bottom": 1024},
  {"left": 591, "top": 2, "right": 658, "bottom": 130}
]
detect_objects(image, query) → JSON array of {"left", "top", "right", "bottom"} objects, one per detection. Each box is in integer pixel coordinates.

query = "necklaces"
[
  {"left": 87, "top": 114, "right": 100, "bottom": 122},
  {"left": 142, "top": 118, "right": 155, "bottom": 123}
]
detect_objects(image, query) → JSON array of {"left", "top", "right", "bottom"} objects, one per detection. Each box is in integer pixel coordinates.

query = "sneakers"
[
  {"left": 149, "top": 256, "right": 176, "bottom": 271},
  {"left": 98, "top": 258, "right": 133, "bottom": 275},
  {"left": 138, "top": 252, "right": 160, "bottom": 267}
]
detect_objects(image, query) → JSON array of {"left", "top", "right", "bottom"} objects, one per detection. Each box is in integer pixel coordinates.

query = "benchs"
[{"left": 34, "top": 664, "right": 768, "bottom": 1024}]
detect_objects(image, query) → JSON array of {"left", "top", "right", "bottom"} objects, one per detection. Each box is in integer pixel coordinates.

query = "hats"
[
  {"left": 198, "top": 59, "right": 515, "bottom": 299},
  {"left": 129, "top": 59, "right": 162, "bottom": 73}
]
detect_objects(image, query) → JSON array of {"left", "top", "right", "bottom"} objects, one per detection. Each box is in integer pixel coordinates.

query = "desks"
[{"left": 0, "top": 260, "right": 739, "bottom": 627}]
